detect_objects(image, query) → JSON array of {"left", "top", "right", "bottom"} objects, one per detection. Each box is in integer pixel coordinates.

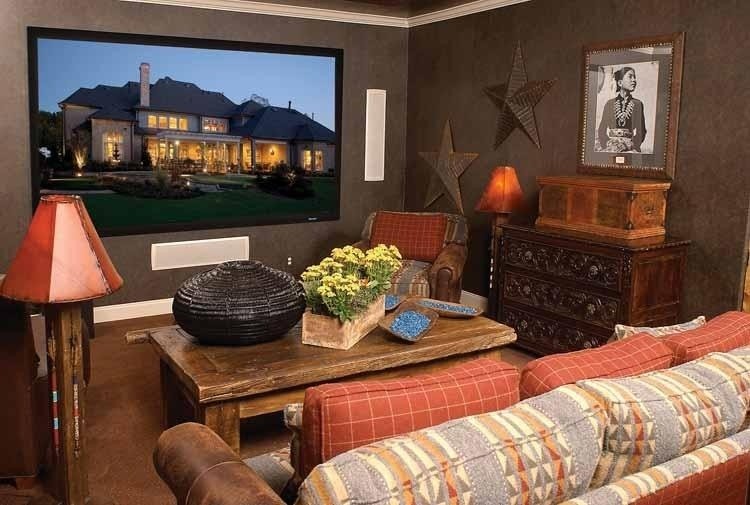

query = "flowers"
[{"left": 297, "top": 239, "right": 405, "bottom": 317}]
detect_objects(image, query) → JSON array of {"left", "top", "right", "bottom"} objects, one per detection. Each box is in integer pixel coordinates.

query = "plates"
[
  {"left": 406, "top": 296, "right": 484, "bottom": 318},
  {"left": 385, "top": 296, "right": 407, "bottom": 310},
  {"left": 377, "top": 301, "right": 440, "bottom": 344}
]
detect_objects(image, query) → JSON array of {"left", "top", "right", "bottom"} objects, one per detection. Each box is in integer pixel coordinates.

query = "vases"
[{"left": 298, "top": 291, "right": 388, "bottom": 351}]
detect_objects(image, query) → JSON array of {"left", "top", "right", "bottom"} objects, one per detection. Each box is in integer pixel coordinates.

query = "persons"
[{"left": 594, "top": 65, "right": 649, "bottom": 153}]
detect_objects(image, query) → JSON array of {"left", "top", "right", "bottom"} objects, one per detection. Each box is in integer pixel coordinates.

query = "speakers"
[
  {"left": 363, "top": 89, "right": 388, "bottom": 182},
  {"left": 151, "top": 237, "right": 249, "bottom": 270}
]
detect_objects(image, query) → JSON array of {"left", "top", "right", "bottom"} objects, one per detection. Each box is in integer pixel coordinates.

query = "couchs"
[{"left": 143, "top": 306, "right": 750, "bottom": 505}]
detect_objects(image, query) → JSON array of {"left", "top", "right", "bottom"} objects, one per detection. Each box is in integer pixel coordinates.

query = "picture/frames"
[{"left": 571, "top": 26, "right": 691, "bottom": 183}]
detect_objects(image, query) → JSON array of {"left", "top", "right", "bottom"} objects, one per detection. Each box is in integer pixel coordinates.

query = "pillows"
[
  {"left": 655, "top": 306, "right": 750, "bottom": 374},
  {"left": 610, "top": 310, "right": 709, "bottom": 348},
  {"left": 516, "top": 326, "right": 677, "bottom": 400},
  {"left": 277, "top": 399, "right": 308, "bottom": 502},
  {"left": 366, "top": 209, "right": 449, "bottom": 260},
  {"left": 294, "top": 350, "right": 524, "bottom": 481}
]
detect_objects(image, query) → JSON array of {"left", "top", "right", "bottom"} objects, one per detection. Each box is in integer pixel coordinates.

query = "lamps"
[
  {"left": 474, "top": 162, "right": 534, "bottom": 316},
  {"left": 0, "top": 194, "right": 126, "bottom": 504}
]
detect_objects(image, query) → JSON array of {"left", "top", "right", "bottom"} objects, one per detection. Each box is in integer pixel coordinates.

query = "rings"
[{"left": 616, "top": 145, "right": 620, "bottom": 148}]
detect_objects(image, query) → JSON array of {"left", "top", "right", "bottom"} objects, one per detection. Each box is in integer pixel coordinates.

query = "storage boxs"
[{"left": 528, "top": 169, "right": 675, "bottom": 244}]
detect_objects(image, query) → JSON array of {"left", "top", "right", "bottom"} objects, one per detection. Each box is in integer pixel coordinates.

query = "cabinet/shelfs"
[
  {"left": 0, "top": 277, "right": 95, "bottom": 491},
  {"left": 496, "top": 217, "right": 694, "bottom": 364}
]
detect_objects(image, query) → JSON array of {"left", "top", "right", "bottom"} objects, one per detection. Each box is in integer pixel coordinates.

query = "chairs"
[{"left": 346, "top": 206, "right": 473, "bottom": 310}]
